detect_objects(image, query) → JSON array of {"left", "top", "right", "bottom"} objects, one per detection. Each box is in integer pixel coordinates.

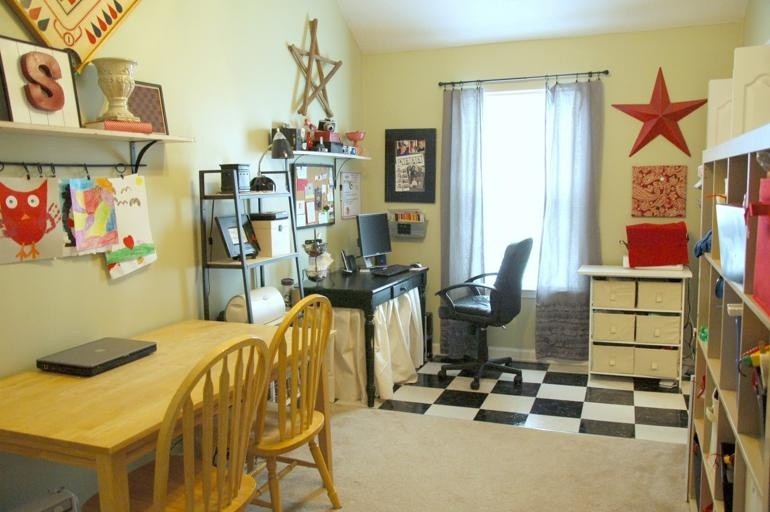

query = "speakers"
[
  {"left": 345, "top": 254, "right": 356, "bottom": 272},
  {"left": 375, "top": 254, "right": 386, "bottom": 266}
]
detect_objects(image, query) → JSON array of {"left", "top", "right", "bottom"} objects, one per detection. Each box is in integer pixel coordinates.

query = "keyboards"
[{"left": 372, "top": 263, "right": 410, "bottom": 277}]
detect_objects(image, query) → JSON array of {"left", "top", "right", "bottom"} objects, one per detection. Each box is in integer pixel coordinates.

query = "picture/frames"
[
  {"left": 4, "top": 0, "right": 139, "bottom": 77},
  {"left": 341, "top": 171, "right": 361, "bottom": 219},
  {"left": 384, "top": 128, "right": 436, "bottom": 204},
  {"left": 1, "top": 35, "right": 84, "bottom": 129},
  {"left": 290, "top": 163, "right": 335, "bottom": 229},
  {"left": 126, "top": 79, "right": 169, "bottom": 135},
  {"left": 215, "top": 213, "right": 262, "bottom": 261}
]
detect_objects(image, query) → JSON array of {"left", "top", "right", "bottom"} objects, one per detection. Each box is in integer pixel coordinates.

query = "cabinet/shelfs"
[
  {"left": 199, "top": 169, "right": 306, "bottom": 326},
  {"left": 578, "top": 264, "right": 693, "bottom": 389},
  {"left": 686, "top": 122, "right": 770, "bottom": 512}
]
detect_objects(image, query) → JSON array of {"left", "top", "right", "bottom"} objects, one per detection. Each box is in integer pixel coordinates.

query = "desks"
[
  {"left": 0, "top": 319, "right": 337, "bottom": 512},
  {"left": 293, "top": 263, "right": 430, "bottom": 408}
]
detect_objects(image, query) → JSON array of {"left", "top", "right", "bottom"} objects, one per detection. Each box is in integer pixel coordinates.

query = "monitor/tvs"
[{"left": 356, "top": 212, "right": 393, "bottom": 268}]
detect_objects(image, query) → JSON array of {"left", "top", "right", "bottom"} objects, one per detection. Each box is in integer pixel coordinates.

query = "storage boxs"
[{"left": 247, "top": 211, "right": 291, "bottom": 258}]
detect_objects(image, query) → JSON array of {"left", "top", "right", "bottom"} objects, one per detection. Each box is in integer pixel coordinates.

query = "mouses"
[{"left": 410, "top": 262, "right": 422, "bottom": 268}]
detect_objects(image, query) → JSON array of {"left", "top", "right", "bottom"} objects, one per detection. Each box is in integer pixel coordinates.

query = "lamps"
[{"left": 249, "top": 128, "right": 294, "bottom": 191}]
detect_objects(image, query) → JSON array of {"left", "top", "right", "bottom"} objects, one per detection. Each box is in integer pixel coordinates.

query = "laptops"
[{"left": 37, "top": 337, "right": 156, "bottom": 377}]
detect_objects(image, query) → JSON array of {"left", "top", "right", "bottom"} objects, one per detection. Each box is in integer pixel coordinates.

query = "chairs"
[
  {"left": 193, "top": 292, "right": 342, "bottom": 511},
  {"left": 81, "top": 335, "right": 270, "bottom": 512},
  {"left": 436, "top": 238, "right": 534, "bottom": 390}
]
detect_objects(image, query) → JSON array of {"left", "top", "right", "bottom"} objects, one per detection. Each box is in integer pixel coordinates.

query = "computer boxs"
[{"left": 423, "top": 311, "right": 433, "bottom": 362}]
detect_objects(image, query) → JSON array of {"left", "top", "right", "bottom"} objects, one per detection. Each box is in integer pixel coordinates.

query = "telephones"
[{"left": 341, "top": 249, "right": 353, "bottom": 274}]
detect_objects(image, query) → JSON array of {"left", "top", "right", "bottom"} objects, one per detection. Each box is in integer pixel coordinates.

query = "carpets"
[{"left": 240, "top": 404, "right": 691, "bottom": 512}]
